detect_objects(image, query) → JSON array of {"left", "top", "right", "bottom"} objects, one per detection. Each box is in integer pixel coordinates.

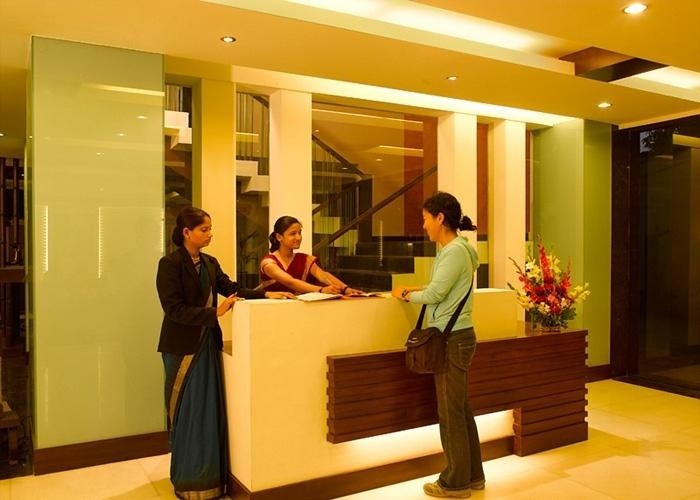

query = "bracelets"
[
  {"left": 342, "top": 286, "right": 349, "bottom": 295},
  {"left": 319, "top": 285, "right": 325, "bottom": 293}
]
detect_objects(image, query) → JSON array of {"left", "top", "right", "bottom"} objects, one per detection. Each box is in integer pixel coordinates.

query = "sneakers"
[
  {"left": 424, "top": 482, "right": 471, "bottom": 497},
  {"left": 471, "top": 484, "right": 485, "bottom": 489}
]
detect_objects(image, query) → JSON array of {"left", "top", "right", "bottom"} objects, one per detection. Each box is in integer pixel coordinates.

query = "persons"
[
  {"left": 391, "top": 191, "right": 485, "bottom": 498},
  {"left": 258, "top": 216, "right": 366, "bottom": 297},
  {"left": 155, "top": 207, "right": 298, "bottom": 500}
]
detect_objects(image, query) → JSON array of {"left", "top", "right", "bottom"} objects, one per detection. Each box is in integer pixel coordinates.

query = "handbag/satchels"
[{"left": 404, "top": 328, "right": 447, "bottom": 375}]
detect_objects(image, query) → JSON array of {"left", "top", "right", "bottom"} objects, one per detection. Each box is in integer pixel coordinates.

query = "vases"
[{"left": 529, "top": 310, "right": 562, "bottom": 332}]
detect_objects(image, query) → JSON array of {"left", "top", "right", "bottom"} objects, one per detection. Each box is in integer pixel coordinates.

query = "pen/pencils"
[
  {"left": 323, "top": 274, "right": 332, "bottom": 285},
  {"left": 232, "top": 292, "right": 237, "bottom": 297}
]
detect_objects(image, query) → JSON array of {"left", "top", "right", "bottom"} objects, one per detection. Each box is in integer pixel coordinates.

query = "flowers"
[{"left": 503, "top": 232, "right": 593, "bottom": 329}]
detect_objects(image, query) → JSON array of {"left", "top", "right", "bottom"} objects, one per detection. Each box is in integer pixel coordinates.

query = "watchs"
[{"left": 402, "top": 289, "right": 410, "bottom": 303}]
